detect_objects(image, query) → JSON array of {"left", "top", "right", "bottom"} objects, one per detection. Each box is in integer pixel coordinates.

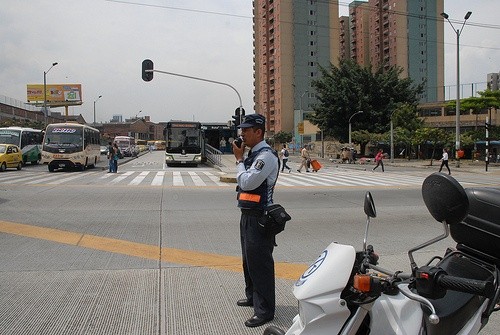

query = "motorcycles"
[{"left": 263, "top": 171, "right": 500, "bottom": 335}]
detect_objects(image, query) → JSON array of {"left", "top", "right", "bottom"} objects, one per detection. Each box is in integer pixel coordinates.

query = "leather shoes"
[
  {"left": 237, "top": 299, "right": 251, "bottom": 306},
  {"left": 244, "top": 312, "right": 273, "bottom": 327}
]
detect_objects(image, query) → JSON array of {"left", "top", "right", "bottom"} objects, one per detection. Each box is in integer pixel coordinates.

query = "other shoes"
[
  {"left": 297, "top": 170, "right": 301, "bottom": 173},
  {"left": 372, "top": 169, "right": 375, "bottom": 172},
  {"left": 289, "top": 168, "right": 291, "bottom": 173},
  {"left": 306, "top": 171, "right": 310, "bottom": 172},
  {"left": 280, "top": 171, "right": 284, "bottom": 173}
]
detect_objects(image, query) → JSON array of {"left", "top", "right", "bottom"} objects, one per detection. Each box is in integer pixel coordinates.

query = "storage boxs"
[{"left": 450, "top": 186, "right": 500, "bottom": 264}]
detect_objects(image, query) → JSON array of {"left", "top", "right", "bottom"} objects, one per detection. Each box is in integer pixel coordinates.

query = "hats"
[{"left": 237, "top": 114, "right": 265, "bottom": 127}]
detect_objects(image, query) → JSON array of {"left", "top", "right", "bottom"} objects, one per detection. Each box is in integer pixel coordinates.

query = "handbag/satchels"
[
  {"left": 114, "top": 155, "right": 118, "bottom": 160},
  {"left": 258, "top": 204, "right": 292, "bottom": 234}
]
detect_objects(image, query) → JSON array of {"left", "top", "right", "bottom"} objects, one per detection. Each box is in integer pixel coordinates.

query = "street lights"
[
  {"left": 43, "top": 62, "right": 58, "bottom": 125},
  {"left": 93, "top": 96, "right": 102, "bottom": 127},
  {"left": 441, "top": 11, "right": 473, "bottom": 167},
  {"left": 292, "top": 83, "right": 309, "bottom": 148},
  {"left": 348, "top": 111, "right": 363, "bottom": 143}
]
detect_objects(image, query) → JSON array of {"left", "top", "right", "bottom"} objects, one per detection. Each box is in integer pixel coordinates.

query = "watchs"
[{"left": 236, "top": 160, "right": 244, "bottom": 165}]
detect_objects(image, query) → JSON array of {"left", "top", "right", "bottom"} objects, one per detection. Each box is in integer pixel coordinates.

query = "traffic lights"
[
  {"left": 232, "top": 114, "right": 240, "bottom": 129},
  {"left": 142, "top": 59, "right": 154, "bottom": 82}
]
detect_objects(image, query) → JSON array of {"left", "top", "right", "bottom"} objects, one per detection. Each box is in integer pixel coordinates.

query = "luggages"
[{"left": 307, "top": 158, "right": 321, "bottom": 172}]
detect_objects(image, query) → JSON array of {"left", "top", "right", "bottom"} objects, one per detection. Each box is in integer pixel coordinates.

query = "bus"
[
  {"left": 0, "top": 126, "right": 45, "bottom": 166},
  {"left": 113, "top": 136, "right": 165, "bottom": 157},
  {"left": 41, "top": 121, "right": 101, "bottom": 172},
  {"left": 162, "top": 120, "right": 203, "bottom": 167}
]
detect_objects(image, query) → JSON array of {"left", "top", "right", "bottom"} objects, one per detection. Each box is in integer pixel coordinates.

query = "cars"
[
  {"left": 100, "top": 146, "right": 108, "bottom": 155},
  {"left": 0, "top": 144, "right": 23, "bottom": 172}
]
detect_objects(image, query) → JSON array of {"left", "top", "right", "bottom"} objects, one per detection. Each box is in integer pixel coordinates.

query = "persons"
[
  {"left": 297, "top": 145, "right": 310, "bottom": 173},
  {"left": 439, "top": 148, "right": 451, "bottom": 175},
  {"left": 220, "top": 137, "right": 226, "bottom": 154},
  {"left": 232, "top": 114, "right": 278, "bottom": 327},
  {"left": 279, "top": 144, "right": 292, "bottom": 173},
  {"left": 372, "top": 150, "right": 385, "bottom": 173},
  {"left": 339, "top": 147, "right": 356, "bottom": 164},
  {"left": 107, "top": 142, "right": 118, "bottom": 173}
]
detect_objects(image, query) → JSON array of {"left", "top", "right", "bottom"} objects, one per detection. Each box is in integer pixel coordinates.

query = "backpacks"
[{"left": 283, "top": 149, "right": 289, "bottom": 157}]
